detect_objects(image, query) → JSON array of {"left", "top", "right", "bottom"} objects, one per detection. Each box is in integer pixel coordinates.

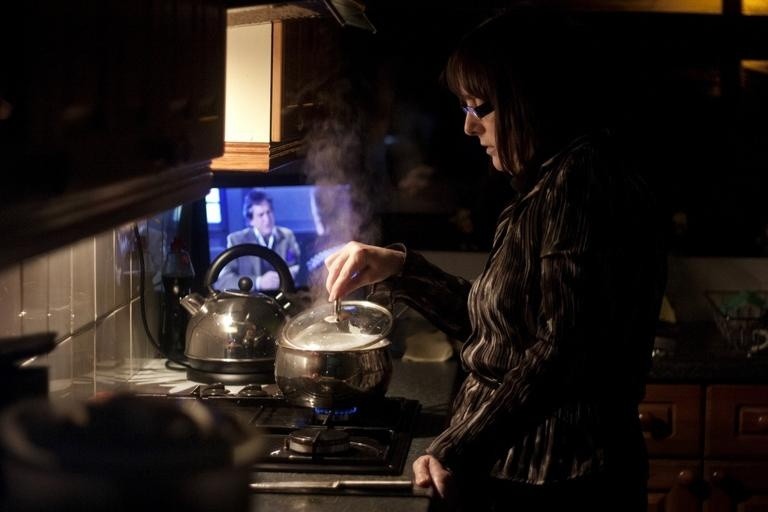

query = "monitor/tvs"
[{"left": 193, "top": 180, "right": 353, "bottom": 295}]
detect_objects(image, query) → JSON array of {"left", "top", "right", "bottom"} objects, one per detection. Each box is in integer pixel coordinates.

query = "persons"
[
  {"left": 218, "top": 192, "right": 306, "bottom": 291},
  {"left": 325, "top": 11, "right": 666, "bottom": 511}
]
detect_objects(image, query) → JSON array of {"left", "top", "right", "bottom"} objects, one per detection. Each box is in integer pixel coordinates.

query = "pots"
[{"left": 273, "top": 296, "right": 395, "bottom": 411}]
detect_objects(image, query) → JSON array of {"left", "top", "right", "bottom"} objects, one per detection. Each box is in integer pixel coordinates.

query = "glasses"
[{"left": 463, "top": 102, "right": 495, "bottom": 123}]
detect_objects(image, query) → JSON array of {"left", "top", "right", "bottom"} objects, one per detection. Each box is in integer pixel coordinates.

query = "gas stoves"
[{"left": 165, "top": 382, "right": 447, "bottom": 493}]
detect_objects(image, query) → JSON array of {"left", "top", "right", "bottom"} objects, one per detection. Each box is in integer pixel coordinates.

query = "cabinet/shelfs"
[{"left": 637, "top": 384, "right": 768, "bottom": 512}]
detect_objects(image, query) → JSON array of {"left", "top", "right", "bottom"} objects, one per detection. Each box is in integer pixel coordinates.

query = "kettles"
[{"left": 178, "top": 243, "right": 297, "bottom": 386}]
